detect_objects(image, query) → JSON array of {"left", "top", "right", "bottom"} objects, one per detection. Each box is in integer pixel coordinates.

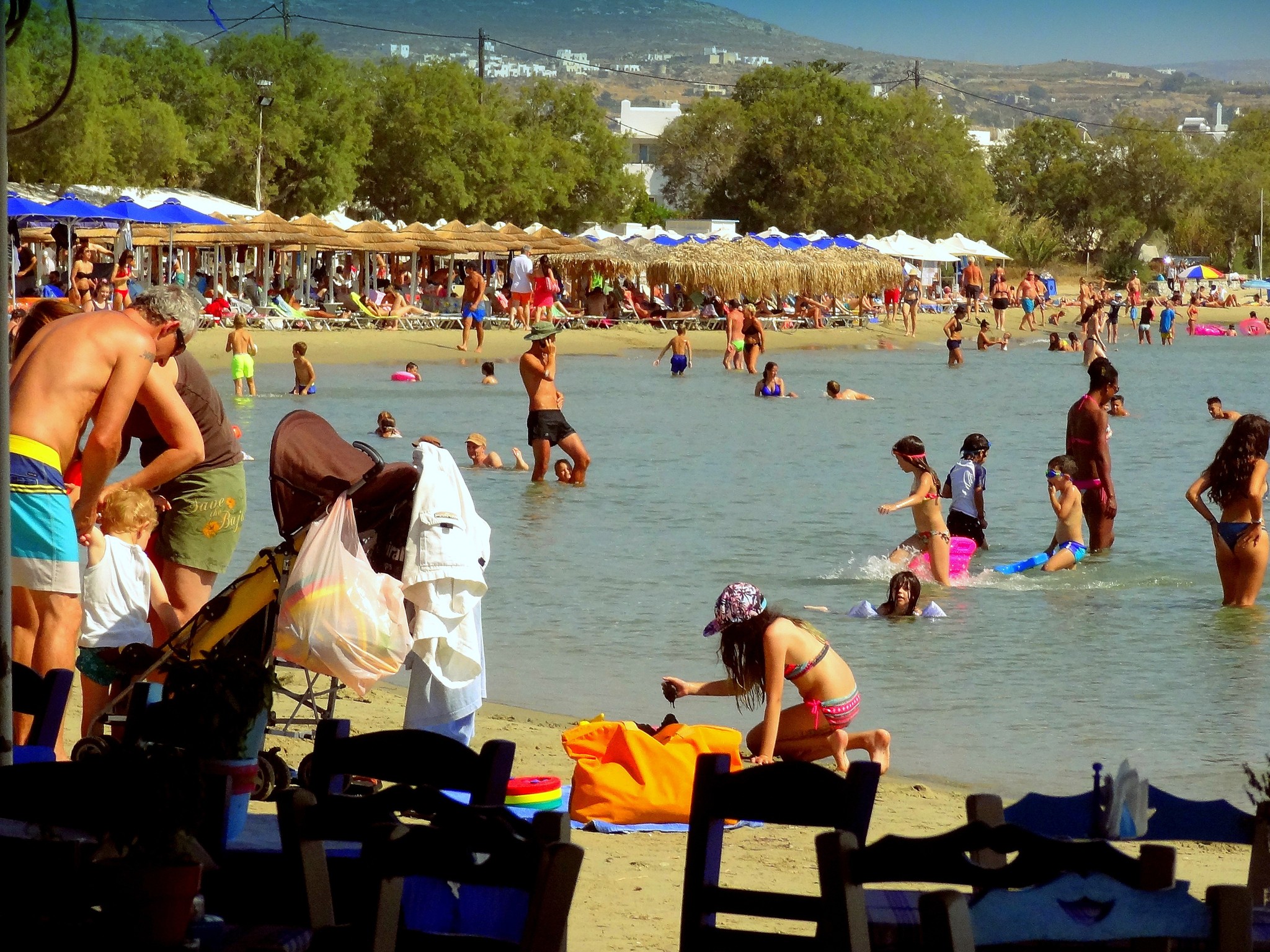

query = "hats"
[
  {"left": 464, "top": 433, "right": 487, "bottom": 447},
  {"left": 908, "top": 269, "right": 918, "bottom": 275},
  {"left": 704, "top": 582, "right": 765, "bottom": 637},
  {"left": 524, "top": 321, "right": 564, "bottom": 340},
  {"left": 1115, "top": 292, "right": 1123, "bottom": 297},
  {"left": 1131, "top": 270, "right": 1138, "bottom": 275},
  {"left": 412, "top": 435, "right": 443, "bottom": 447}
]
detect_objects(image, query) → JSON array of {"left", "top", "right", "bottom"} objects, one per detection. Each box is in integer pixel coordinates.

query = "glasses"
[
  {"left": 1028, "top": 274, "right": 1034, "bottom": 276},
  {"left": 1111, "top": 383, "right": 1120, "bottom": 394},
  {"left": 84, "top": 250, "right": 91, "bottom": 253},
  {"left": 127, "top": 255, "right": 134, "bottom": 259},
  {"left": 1044, "top": 470, "right": 1066, "bottom": 479},
  {"left": 167, "top": 312, "right": 185, "bottom": 358}
]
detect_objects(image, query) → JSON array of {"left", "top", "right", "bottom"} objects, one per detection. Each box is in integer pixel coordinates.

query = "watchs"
[{"left": 1205, "top": 518, "right": 1214, "bottom": 523}]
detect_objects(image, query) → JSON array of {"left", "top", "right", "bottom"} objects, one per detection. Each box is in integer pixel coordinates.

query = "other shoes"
[
  {"left": 975, "top": 317, "right": 982, "bottom": 324},
  {"left": 966, "top": 318, "right": 970, "bottom": 322}
]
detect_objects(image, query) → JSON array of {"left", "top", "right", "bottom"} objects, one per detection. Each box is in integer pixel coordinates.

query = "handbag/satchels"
[
  {"left": 545, "top": 268, "right": 560, "bottom": 292},
  {"left": 275, "top": 489, "right": 413, "bottom": 698},
  {"left": 561, "top": 713, "right": 746, "bottom": 825}
]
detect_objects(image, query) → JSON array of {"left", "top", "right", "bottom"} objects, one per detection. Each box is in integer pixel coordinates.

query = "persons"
[
  {"left": 371, "top": 410, "right": 529, "bottom": 472},
  {"left": 13, "top": 243, "right": 921, "bottom": 339},
  {"left": 1040, "top": 356, "right": 1130, "bottom": 571},
  {"left": 802, "top": 571, "right": 923, "bottom": 616},
  {"left": 226, "top": 311, "right": 315, "bottom": 396},
  {"left": 457, "top": 260, "right": 486, "bottom": 352},
  {"left": 10, "top": 282, "right": 248, "bottom": 761},
  {"left": 941, "top": 255, "right": 1270, "bottom": 366},
  {"left": 876, "top": 433, "right": 991, "bottom": 587},
  {"left": 406, "top": 362, "right": 422, "bottom": 382},
  {"left": 663, "top": 580, "right": 890, "bottom": 776},
  {"left": 520, "top": 320, "right": 590, "bottom": 485},
  {"left": 1185, "top": 396, "right": 1270, "bottom": 606},
  {"left": 480, "top": 361, "right": 499, "bottom": 384},
  {"left": 654, "top": 301, "right": 875, "bottom": 402}
]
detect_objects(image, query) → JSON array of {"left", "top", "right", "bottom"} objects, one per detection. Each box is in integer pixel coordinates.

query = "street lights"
[{"left": 256, "top": 95, "right": 274, "bottom": 213}]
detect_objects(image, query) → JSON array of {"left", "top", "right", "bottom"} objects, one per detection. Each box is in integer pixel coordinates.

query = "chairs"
[
  {"left": 681, "top": 753, "right": 1270, "bottom": 952},
  {"left": 0, "top": 664, "right": 584, "bottom": 952},
  {"left": 196, "top": 280, "right": 985, "bottom": 331}
]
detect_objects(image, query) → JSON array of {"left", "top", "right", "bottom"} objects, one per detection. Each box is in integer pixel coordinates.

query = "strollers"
[{"left": 0, "top": 407, "right": 429, "bottom": 952}]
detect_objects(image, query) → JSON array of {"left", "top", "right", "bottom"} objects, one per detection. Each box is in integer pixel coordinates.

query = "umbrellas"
[
  {"left": 8, "top": 190, "right": 226, "bottom": 285},
  {"left": 1176, "top": 264, "right": 1226, "bottom": 283},
  {"left": 1243, "top": 280, "right": 1270, "bottom": 290},
  {"left": 132, "top": 211, "right": 905, "bottom": 308},
  {"left": 322, "top": 211, "right": 1014, "bottom": 311}
]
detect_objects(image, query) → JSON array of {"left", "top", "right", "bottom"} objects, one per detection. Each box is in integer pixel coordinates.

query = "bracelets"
[{"left": 1249, "top": 518, "right": 1262, "bottom": 524}]
